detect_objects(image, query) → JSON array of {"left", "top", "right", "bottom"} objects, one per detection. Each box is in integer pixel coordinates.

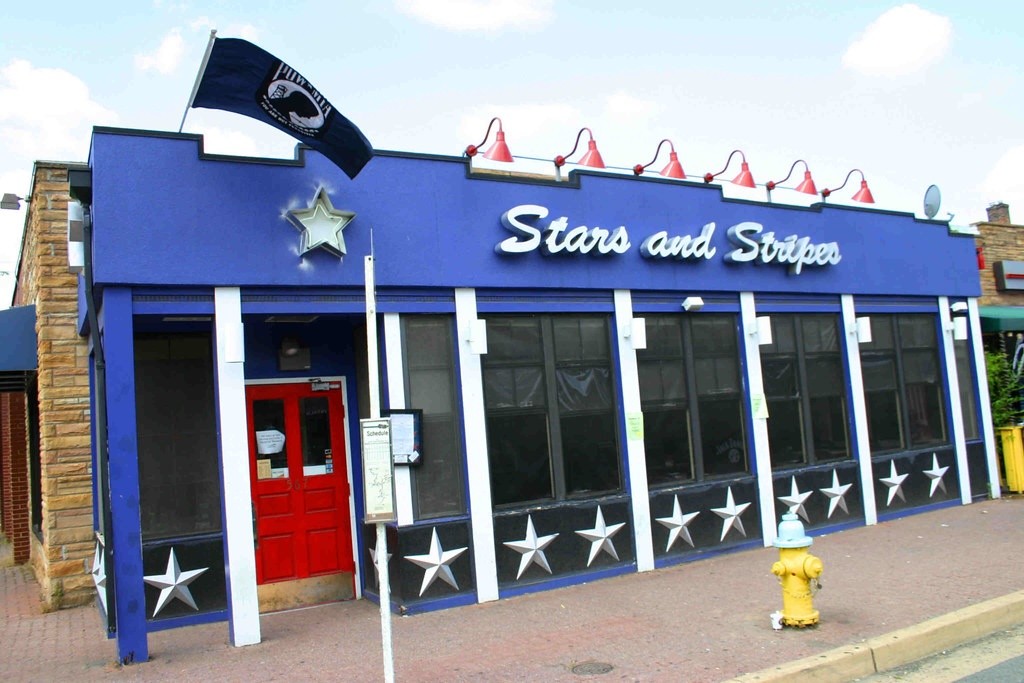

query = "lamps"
[
  {"left": 463, "top": 118, "right": 513, "bottom": 163},
  {"left": 850, "top": 316, "right": 871, "bottom": 343},
  {"left": 705, "top": 150, "right": 756, "bottom": 189},
  {"left": 624, "top": 317, "right": 647, "bottom": 349},
  {"left": 768, "top": 158, "right": 818, "bottom": 195},
  {"left": 556, "top": 126, "right": 605, "bottom": 169},
  {"left": 750, "top": 316, "right": 772, "bottom": 345},
  {"left": 0, "top": 192, "right": 30, "bottom": 211},
  {"left": 823, "top": 169, "right": 875, "bottom": 204},
  {"left": 466, "top": 319, "right": 488, "bottom": 355},
  {"left": 633, "top": 139, "right": 687, "bottom": 180},
  {"left": 945, "top": 317, "right": 967, "bottom": 341},
  {"left": 681, "top": 295, "right": 705, "bottom": 311}
]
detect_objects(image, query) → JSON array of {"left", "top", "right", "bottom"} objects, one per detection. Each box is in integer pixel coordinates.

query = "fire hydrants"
[{"left": 769, "top": 510, "right": 826, "bottom": 629}]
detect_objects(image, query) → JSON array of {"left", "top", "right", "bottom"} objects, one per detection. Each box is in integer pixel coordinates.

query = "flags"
[{"left": 191, "top": 38, "right": 374, "bottom": 181}]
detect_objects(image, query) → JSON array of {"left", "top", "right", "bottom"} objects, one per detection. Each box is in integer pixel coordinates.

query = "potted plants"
[{"left": 984, "top": 351, "right": 1024, "bottom": 493}]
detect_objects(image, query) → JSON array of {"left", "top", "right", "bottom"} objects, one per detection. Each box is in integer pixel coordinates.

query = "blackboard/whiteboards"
[{"left": 359, "top": 417, "right": 397, "bottom": 524}]
